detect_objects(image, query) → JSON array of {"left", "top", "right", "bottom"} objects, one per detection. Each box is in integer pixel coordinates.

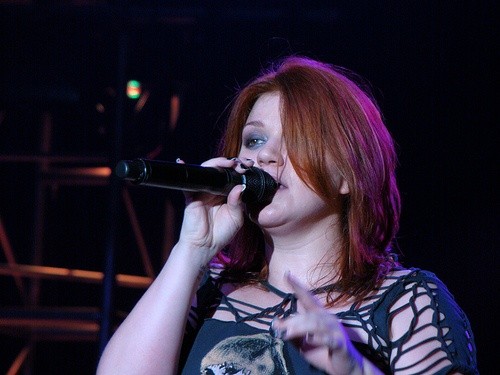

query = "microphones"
[{"left": 116, "top": 158, "right": 278, "bottom": 207}]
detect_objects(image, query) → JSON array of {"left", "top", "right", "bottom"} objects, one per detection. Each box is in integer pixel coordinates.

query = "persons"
[{"left": 94, "top": 56, "right": 478, "bottom": 375}]
